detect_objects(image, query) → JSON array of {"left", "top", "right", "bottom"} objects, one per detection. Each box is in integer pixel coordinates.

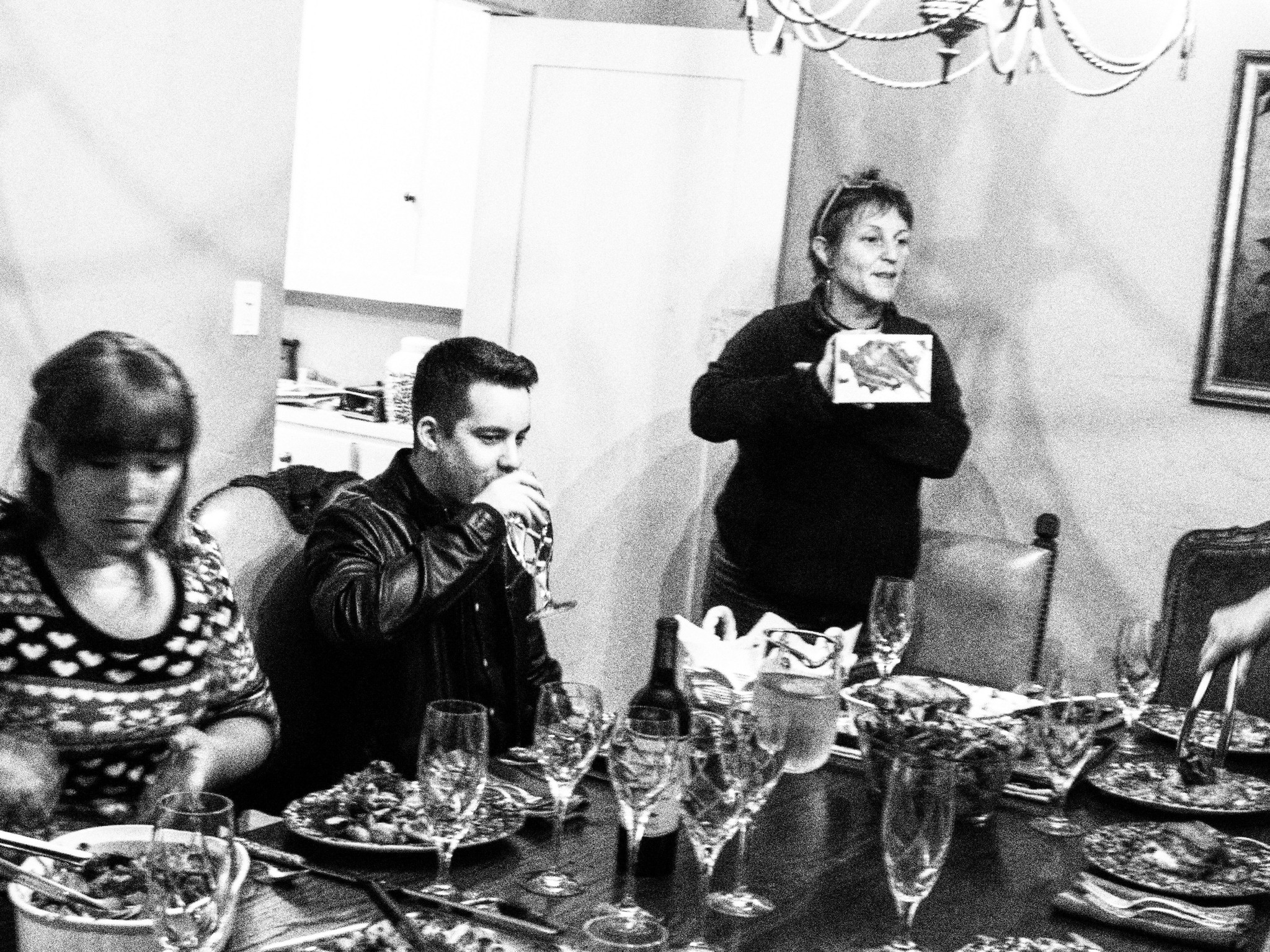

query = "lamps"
[{"left": 739, "top": 0, "right": 1199, "bottom": 99}]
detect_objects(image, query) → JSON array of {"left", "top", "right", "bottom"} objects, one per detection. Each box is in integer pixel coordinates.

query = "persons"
[
  {"left": 0, "top": 329, "right": 282, "bottom": 841},
  {"left": 690, "top": 165, "right": 972, "bottom": 685},
  {"left": 1192, "top": 587, "right": 1270, "bottom": 693},
  {"left": 302, "top": 337, "right": 573, "bottom": 781}
]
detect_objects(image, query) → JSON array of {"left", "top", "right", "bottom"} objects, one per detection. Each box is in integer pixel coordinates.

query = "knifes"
[{"left": 234, "top": 836, "right": 359, "bottom": 882}]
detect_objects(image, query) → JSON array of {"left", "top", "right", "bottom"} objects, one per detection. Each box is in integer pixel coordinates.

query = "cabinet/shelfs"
[
  {"left": 267, "top": 418, "right": 411, "bottom": 481},
  {"left": 282, "top": 0, "right": 488, "bottom": 309}
]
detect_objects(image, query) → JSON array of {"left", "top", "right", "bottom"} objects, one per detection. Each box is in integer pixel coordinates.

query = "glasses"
[{"left": 817, "top": 174, "right": 905, "bottom": 234}]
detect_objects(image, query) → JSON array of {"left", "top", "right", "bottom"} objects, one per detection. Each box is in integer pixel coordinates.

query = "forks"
[
  {"left": 251, "top": 859, "right": 311, "bottom": 880},
  {"left": 1080, "top": 882, "right": 1244, "bottom": 931},
  {"left": 494, "top": 783, "right": 555, "bottom": 809}
]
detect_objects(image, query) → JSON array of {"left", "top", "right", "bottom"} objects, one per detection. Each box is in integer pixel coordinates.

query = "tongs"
[
  {"left": 1175, "top": 652, "right": 1240, "bottom": 768},
  {"left": 0, "top": 830, "right": 145, "bottom": 919},
  {"left": 360, "top": 876, "right": 558, "bottom": 952}
]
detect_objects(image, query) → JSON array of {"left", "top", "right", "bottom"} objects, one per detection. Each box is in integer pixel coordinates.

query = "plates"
[
  {"left": 1137, "top": 703, "right": 1270, "bottom": 752},
  {"left": 1086, "top": 755, "right": 1270, "bottom": 815},
  {"left": 283, "top": 781, "right": 525, "bottom": 850},
  {"left": 1081, "top": 822, "right": 1270, "bottom": 897}
]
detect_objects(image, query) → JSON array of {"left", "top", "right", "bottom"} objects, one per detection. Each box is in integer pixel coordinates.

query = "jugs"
[{"left": 753, "top": 629, "right": 843, "bottom": 773}]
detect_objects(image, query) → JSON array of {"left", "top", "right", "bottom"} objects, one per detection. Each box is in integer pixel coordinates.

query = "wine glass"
[
  {"left": 1114, "top": 616, "right": 1161, "bottom": 755},
  {"left": 419, "top": 699, "right": 489, "bottom": 897},
  {"left": 504, "top": 468, "right": 577, "bottom": 621},
  {"left": 582, "top": 706, "right": 692, "bottom": 948},
  {"left": 709, "top": 699, "right": 790, "bottom": 918},
  {"left": 1030, "top": 689, "right": 1098, "bottom": 836},
  {"left": 672, "top": 709, "right": 745, "bottom": 952},
  {"left": 880, "top": 755, "right": 955, "bottom": 952},
  {"left": 513, "top": 682, "right": 602, "bottom": 898}
]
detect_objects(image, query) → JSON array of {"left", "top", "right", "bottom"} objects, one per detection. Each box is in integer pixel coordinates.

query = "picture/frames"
[{"left": 1190, "top": 48, "right": 1269, "bottom": 413}]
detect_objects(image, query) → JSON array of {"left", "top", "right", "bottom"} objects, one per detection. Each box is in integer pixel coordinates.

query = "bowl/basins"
[
  {"left": 840, "top": 674, "right": 1031, "bottom": 723},
  {"left": 854, "top": 707, "right": 1015, "bottom": 828},
  {"left": 9, "top": 825, "right": 250, "bottom": 952}
]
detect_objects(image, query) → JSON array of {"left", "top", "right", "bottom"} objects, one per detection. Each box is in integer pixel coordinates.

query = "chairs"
[
  {"left": 1150, "top": 518, "right": 1270, "bottom": 722},
  {"left": 902, "top": 511, "right": 1061, "bottom": 691}
]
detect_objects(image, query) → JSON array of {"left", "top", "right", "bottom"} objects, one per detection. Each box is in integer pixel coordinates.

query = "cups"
[
  {"left": 158, "top": 791, "right": 234, "bottom": 952},
  {"left": 869, "top": 576, "right": 914, "bottom": 677}
]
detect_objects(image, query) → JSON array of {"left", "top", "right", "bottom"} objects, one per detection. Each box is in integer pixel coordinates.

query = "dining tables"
[{"left": 0, "top": 663, "right": 1270, "bottom": 952}]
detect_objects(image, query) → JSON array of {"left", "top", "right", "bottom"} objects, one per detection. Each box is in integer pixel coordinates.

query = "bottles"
[{"left": 612, "top": 617, "right": 688, "bottom": 911}]
